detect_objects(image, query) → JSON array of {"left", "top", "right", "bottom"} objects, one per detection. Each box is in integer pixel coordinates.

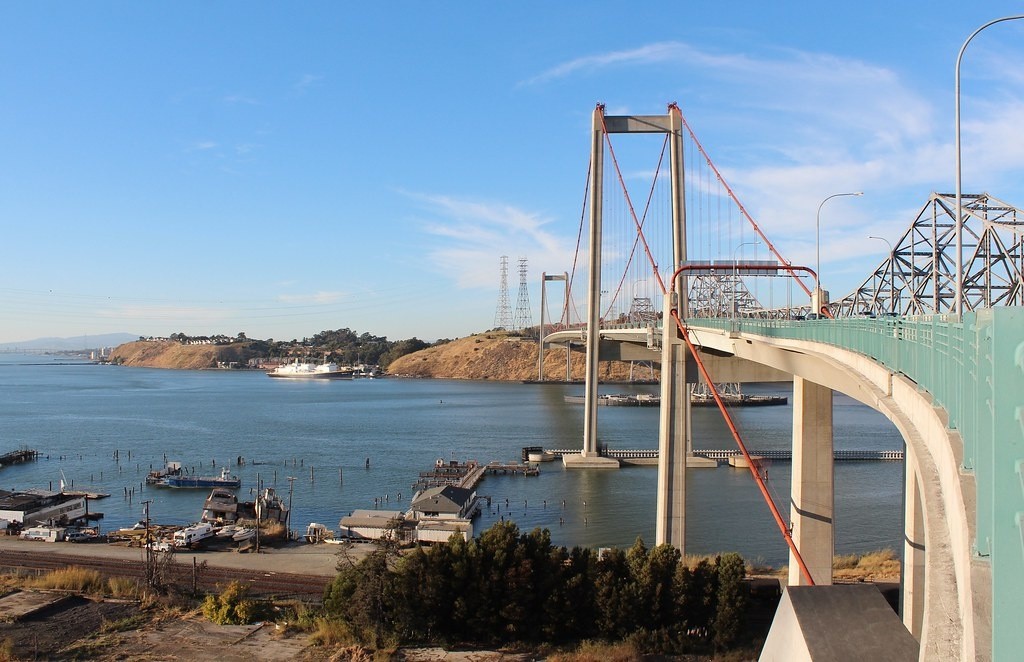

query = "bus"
[{"left": 174, "top": 522, "right": 213, "bottom": 547}]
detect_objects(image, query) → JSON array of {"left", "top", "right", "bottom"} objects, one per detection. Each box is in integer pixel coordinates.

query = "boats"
[
  {"left": 143, "top": 463, "right": 182, "bottom": 486},
  {"left": 302, "top": 523, "right": 336, "bottom": 545},
  {"left": 173, "top": 487, "right": 290, "bottom": 542},
  {"left": 155, "top": 475, "right": 242, "bottom": 491},
  {"left": 601, "top": 391, "right": 789, "bottom": 407},
  {"left": 266, "top": 358, "right": 380, "bottom": 379}
]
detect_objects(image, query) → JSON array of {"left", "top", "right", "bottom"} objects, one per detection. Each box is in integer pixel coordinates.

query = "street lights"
[
  {"left": 631, "top": 279, "right": 647, "bottom": 299},
  {"left": 732, "top": 240, "right": 762, "bottom": 332},
  {"left": 662, "top": 263, "right": 681, "bottom": 290},
  {"left": 813, "top": 190, "right": 865, "bottom": 320}
]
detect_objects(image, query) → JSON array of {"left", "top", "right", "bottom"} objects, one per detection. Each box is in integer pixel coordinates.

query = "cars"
[{"left": 64, "top": 533, "right": 92, "bottom": 543}]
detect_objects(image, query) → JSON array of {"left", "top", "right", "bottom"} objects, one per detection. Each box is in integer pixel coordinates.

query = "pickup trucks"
[{"left": 145, "top": 542, "right": 169, "bottom": 553}]
